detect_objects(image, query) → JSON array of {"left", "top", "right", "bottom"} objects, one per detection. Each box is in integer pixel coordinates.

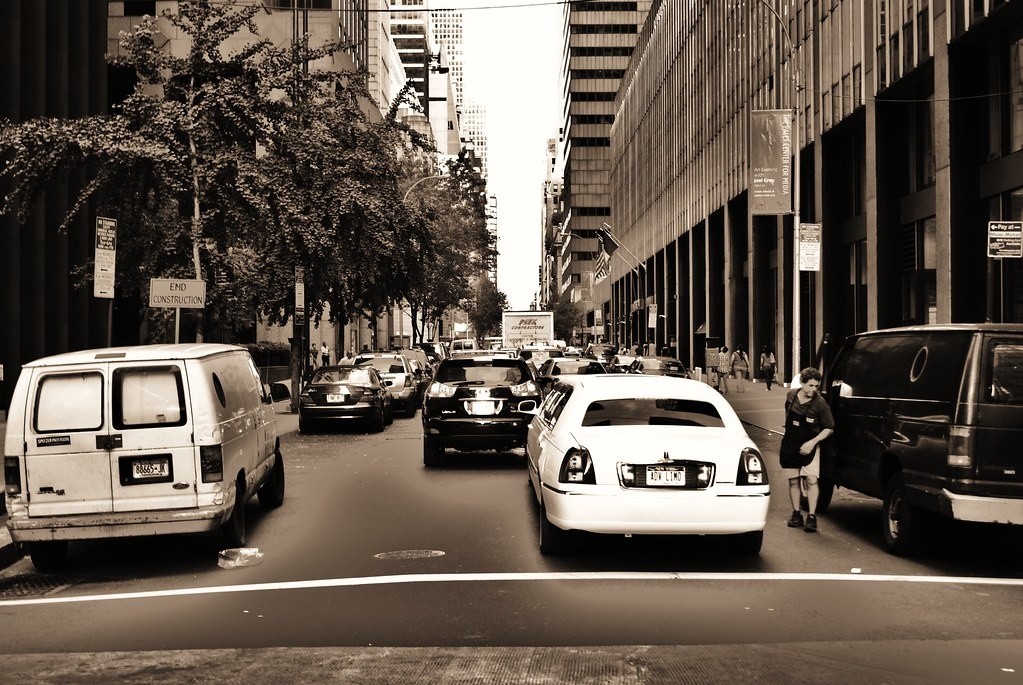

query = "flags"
[
  {"left": 602, "top": 227, "right": 619, "bottom": 267},
  {"left": 595, "top": 237, "right": 612, "bottom": 286}
]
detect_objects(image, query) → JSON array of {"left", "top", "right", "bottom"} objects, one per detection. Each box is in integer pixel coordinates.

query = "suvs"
[{"left": 419, "top": 353, "right": 552, "bottom": 466}]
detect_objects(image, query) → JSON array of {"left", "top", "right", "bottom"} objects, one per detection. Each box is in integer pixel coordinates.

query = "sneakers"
[
  {"left": 788, "top": 511, "right": 803, "bottom": 527},
  {"left": 805, "top": 514, "right": 816, "bottom": 532}
]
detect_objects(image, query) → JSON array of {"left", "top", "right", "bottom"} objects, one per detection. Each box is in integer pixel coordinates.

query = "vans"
[
  {"left": 813, "top": 321, "right": 1023, "bottom": 555},
  {"left": 1, "top": 343, "right": 291, "bottom": 574}
]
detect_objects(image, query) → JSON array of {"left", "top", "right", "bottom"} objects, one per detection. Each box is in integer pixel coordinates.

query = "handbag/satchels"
[{"left": 769, "top": 354, "right": 776, "bottom": 363}]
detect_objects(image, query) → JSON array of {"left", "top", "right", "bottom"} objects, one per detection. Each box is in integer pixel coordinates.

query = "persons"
[
  {"left": 730, "top": 345, "right": 750, "bottom": 394},
  {"left": 628, "top": 348, "right": 644, "bottom": 374},
  {"left": 712, "top": 346, "right": 730, "bottom": 395},
  {"left": 661, "top": 344, "right": 671, "bottom": 358},
  {"left": 631, "top": 340, "right": 656, "bottom": 357},
  {"left": 346, "top": 345, "right": 354, "bottom": 359},
  {"left": 320, "top": 341, "right": 330, "bottom": 366},
  {"left": 310, "top": 343, "right": 319, "bottom": 369},
  {"left": 358, "top": 343, "right": 372, "bottom": 355},
  {"left": 618, "top": 343, "right": 630, "bottom": 356},
  {"left": 583, "top": 339, "right": 594, "bottom": 352},
  {"left": 760, "top": 345, "right": 776, "bottom": 391},
  {"left": 780, "top": 368, "right": 833, "bottom": 533}
]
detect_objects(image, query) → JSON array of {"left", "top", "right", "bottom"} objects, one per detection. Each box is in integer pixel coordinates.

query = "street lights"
[{"left": 559, "top": 233, "right": 597, "bottom": 343}]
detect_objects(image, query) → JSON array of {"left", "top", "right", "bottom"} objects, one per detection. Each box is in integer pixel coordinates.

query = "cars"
[
  {"left": 345, "top": 336, "right": 691, "bottom": 412},
  {"left": 517, "top": 372, "right": 772, "bottom": 564},
  {"left": 298, "top": 364, "right": 395, "bottom": 434}
]
